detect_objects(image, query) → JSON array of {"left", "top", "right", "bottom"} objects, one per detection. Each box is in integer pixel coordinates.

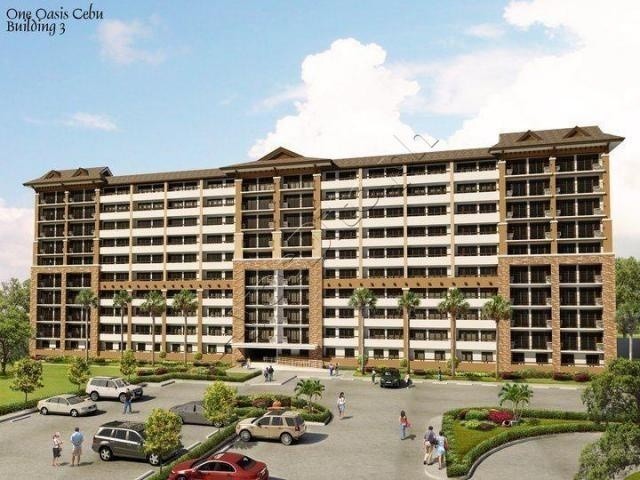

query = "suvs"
[
  {"left": 236, "top": 411, "right": 307, "bottom": 446},
  {"left": 380, "top": 367, "right": 401, "bottom": 387},
  {"left": 86, "top": 375, "right": 143, "bottom": 402}
]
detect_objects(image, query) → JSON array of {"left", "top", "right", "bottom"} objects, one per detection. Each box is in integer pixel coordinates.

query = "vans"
[{"left": 93, "top": 419, "right": 183, "bottom": 465}]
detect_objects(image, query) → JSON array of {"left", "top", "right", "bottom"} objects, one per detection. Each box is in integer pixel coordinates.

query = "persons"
[
  {"left": 51, "top": 431, "right": 64, "bottom": 466},
  {"left": 264, "top": 366, "right": 269, "bottom": 383},
  {"left": 268, "top": 365, "right": 274, "bottom": 383},
  {"left": 336, "top": 391, "right": 346, "bottom": 419},
  {"left": 247, "top": 356, "right": 251, "bottom": 366},
  {"left": 335, "top": 362, "right": 339, "bottom": 376},
  {"left": 399, "top": 410, "right": 408, "bottom": 441},
  {"left": 434, "top": 431, "right": 448, "bottom": 470},
  {"left": 371, "top": 368, "right": 377, "bottom": 383},
  {"left": 68, "top": 426, "right": 84, "bottom": 468},
  {"left": 422, "top": 426, "right": 436, "bottom": 465},
  {"left": 403, "top": 371, "right": 410, "bottom": 392},
  {"left": 122, "top": 388, "right": 134, "bottom": 414},
  {"left": 438, "top": 367, "right": 442, "bottom": 381},
  {"left": 329, "top": 362, "right": 334, "bottom": 376}
]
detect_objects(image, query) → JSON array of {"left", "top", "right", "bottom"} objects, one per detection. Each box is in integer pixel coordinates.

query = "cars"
[
  {"left": 170, "top": 401, "right": 235, "bottom": 427},
  {"left": 168, "top": 452, "right": 269, "bottom": 479},
  {"left": 38, "top": 393, "right": 97, "bottom": 417}
]
